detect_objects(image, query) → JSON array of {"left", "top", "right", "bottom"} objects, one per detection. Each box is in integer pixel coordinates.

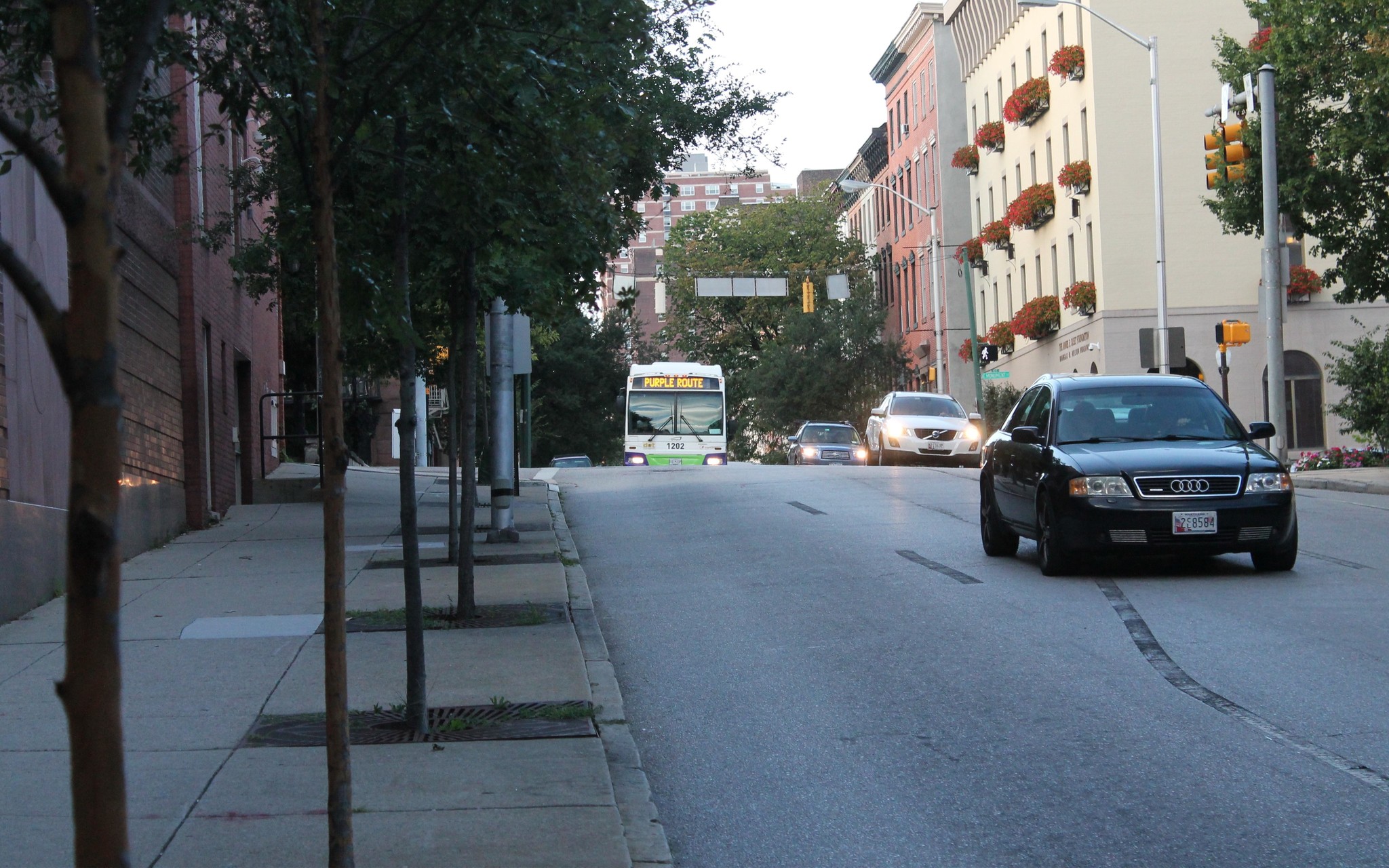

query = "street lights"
[
  {"left": 1017, "top": 0, "right": 1170, "bottom": 375},
  {"left": 839, "top": 179, "right": 945, "bottom": 394}
]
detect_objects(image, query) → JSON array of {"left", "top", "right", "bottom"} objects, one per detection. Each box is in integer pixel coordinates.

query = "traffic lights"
[
  {"left": 928, "top": 366, "right": 936, "bottom": 382},
  {"left": 1203, "top": 131, "right": 1230, "bottom": 190},
  {"left": 1215, "top": 323, "right": 1231, "bottom": 344},
  {"left": 980, "top": 345, "right": 999, "bottom": 362},
  {"left": 1223, "top": 119, "right": 1252, "bottom": 184}
]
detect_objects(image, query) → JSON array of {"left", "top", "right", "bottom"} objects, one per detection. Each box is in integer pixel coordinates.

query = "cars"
[
  {"left": 785, "top": 419, "right": 867, "bottom": 466},
  {"left": 549, "top": 453, "right": 594, "bottom": 468},
  {"left": 863, "top": 391, "right": 982, "bottom": 468},
  {"left": 979, "top": 373, "right": 1298, "bottom": 576}
]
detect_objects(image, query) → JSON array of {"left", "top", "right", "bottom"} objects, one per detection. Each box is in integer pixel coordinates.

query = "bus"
[{"left": 616, "top": 361, "right": 736, "bottom": 467}]
